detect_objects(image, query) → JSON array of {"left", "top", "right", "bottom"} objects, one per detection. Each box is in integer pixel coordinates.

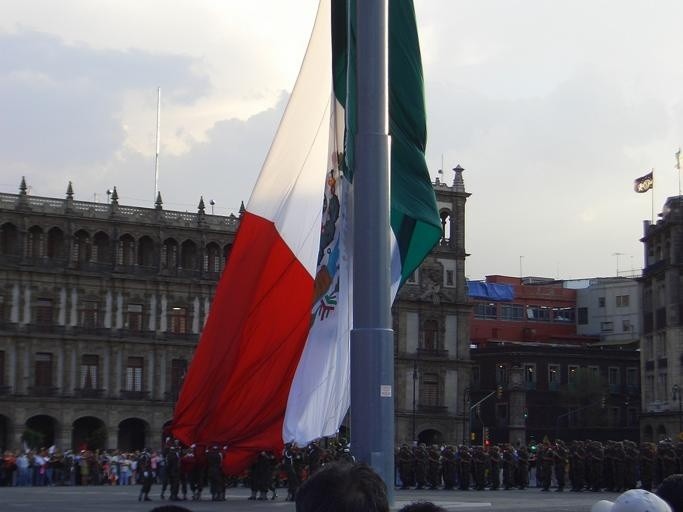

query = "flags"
[
  {"left": 168, "top": 0, "right": 443, "bottom": 474},
  {"left": 633, "top": 171, "right": 652, "bottom": 193}
]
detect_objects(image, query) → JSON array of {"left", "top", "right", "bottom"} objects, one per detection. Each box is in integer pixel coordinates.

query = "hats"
[{"left": 591, "top": 489, "right": 672, "bottom": 512}]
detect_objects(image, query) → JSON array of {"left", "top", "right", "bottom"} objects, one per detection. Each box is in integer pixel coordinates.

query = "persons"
[
  {"left": 295, "top": 459, "right": 449, "bottom": 511},
  {"left": 394, "top": 438, "right": 683, "bottom": 494},
  {"left": 593, "top": 474, "right": 682, "bottom": 511},
  {"left": 1, "top": 437, "right": 351, "bottom": 503}
]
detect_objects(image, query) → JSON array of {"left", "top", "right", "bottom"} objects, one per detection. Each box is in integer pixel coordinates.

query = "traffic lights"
[
  {"left": 497, "top": 385, "right": 503, "bottom": 401},
  {"left": 528, "top": 434, "right": 536, "bottom": 454},
  {"left": 522, "top": 404, "right": 527, "bottom": 418},
  {"left": 483, "top": 428, "right": 489, "bottom": 445}
]
